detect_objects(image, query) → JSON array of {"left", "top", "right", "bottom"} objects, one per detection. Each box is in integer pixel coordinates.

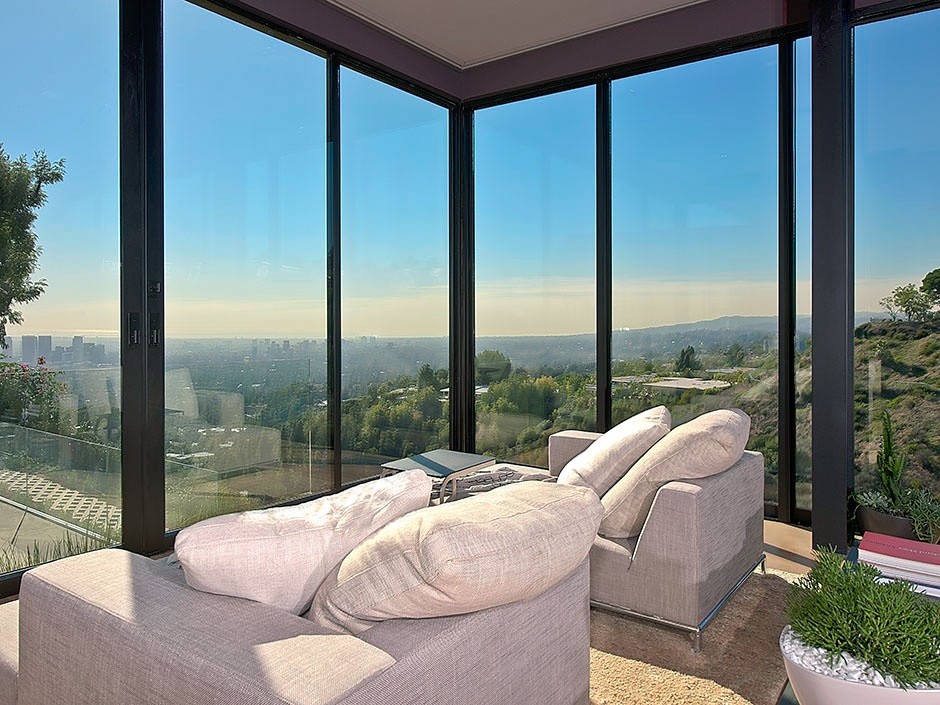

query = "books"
[{"left": 839, "top": 531, "right": 940, "bottom": 598}]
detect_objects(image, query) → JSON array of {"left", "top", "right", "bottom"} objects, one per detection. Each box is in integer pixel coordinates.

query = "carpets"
[{"left": 589, "top": 565, "right": 822, "bottom": 705}]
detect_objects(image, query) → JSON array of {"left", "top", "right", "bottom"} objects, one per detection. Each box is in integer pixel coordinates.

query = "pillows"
[
  {"left": 174, "top": 468, "right": 432, "bottom": 616},
  {"left": 557, "top": 405, "right": 672, "bottom": 499}
]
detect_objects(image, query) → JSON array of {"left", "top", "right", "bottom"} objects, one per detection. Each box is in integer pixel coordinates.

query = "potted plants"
[
  {"left": 847, "top": 408, "right": 940, "bottom": 545},
  {"left": 778, "top": 544, "right": 940, "bottom": 705}
]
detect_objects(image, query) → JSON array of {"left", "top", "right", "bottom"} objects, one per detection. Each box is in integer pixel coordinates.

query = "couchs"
[
  {"left": 0, "top": 480, "right": 604, "bottom": 705},
  {"left": 429, "top": 407, "right": 767, "bottom": 652}
]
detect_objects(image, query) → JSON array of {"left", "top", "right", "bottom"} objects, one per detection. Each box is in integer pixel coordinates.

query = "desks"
[{"left": 381, "top": 449, "right": 496, "bottom": 504}]
